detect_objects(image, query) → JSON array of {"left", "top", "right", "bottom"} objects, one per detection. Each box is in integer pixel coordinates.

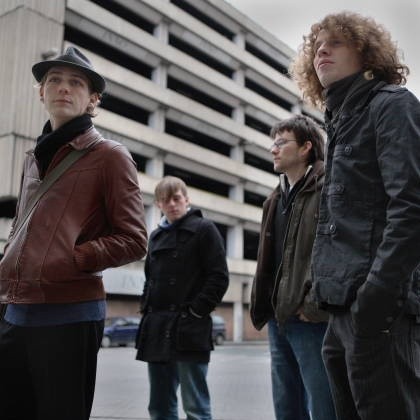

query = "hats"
[{"left": 32, "top": 47, "right": 106, "bottom": 93}]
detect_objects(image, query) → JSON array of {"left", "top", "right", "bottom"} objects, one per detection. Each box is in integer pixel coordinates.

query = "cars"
[
  {"left": 209, "top": 316, "right": 227, "bottom": 345},
  {"left": 101, "top": 316, "right": 140, "bottom": 348}
]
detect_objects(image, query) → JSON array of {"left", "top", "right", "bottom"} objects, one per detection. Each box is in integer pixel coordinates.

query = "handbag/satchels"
[{"left": 136, "top": 311, "right": 180, "bottom": 364}]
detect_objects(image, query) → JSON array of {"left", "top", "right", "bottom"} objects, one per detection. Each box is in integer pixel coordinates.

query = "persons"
[
  {"left": 133, "top": 176, "right": 230, "bottom": 420},
  {"left": 0, "top": 45, "right": 148, "bottom": 420},
  {"left": 249, "top": 113, "right": 336, "bottom": 420},
  {"left": 287, "top": 9, "right": 420, "bottom": 420}
]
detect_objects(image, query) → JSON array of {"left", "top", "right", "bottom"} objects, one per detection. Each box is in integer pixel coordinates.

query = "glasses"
[{"left": 272, "top": 138, "right": 306, "bottom": 148}]
